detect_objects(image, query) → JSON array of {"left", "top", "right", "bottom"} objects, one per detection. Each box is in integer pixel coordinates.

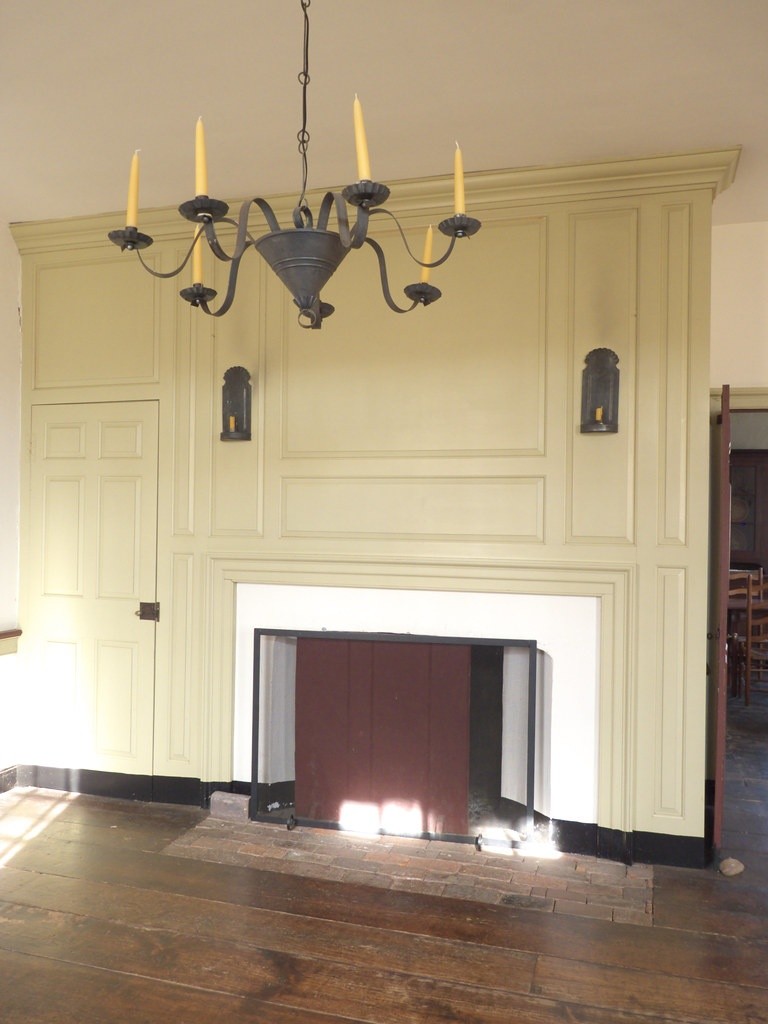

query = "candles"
[
  {"left": 353, "top": 93, "right": 370, "bottom": 181},
  {"left": 194, "top": 116, "right": 208, "bottom": 196},
  {"left": 454, "top": 140, "right": 465, "bottom": 215},
  {"left": 421, "top": 225, "right": 433, "bottom": 284},
  {"left": 192, "top": 225, "right": 202, "bottom": 284},
  {"left": 126, "top": 150, "right": 140, "bottom": 228},
  {"left": 230, "top": 416, "right": 236, "bottom": 432},
  {"left": 596, "top": 406, "right": 603, "bottom": 421}
]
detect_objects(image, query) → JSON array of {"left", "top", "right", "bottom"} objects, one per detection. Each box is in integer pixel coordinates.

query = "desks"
[{"left": 728, "top": 598, "right": 768, "bottom": 696}]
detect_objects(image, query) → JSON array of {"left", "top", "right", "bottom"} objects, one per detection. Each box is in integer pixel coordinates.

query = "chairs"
[{"left": 728, "top": 568, "right": 768, "bottom": 705}]
